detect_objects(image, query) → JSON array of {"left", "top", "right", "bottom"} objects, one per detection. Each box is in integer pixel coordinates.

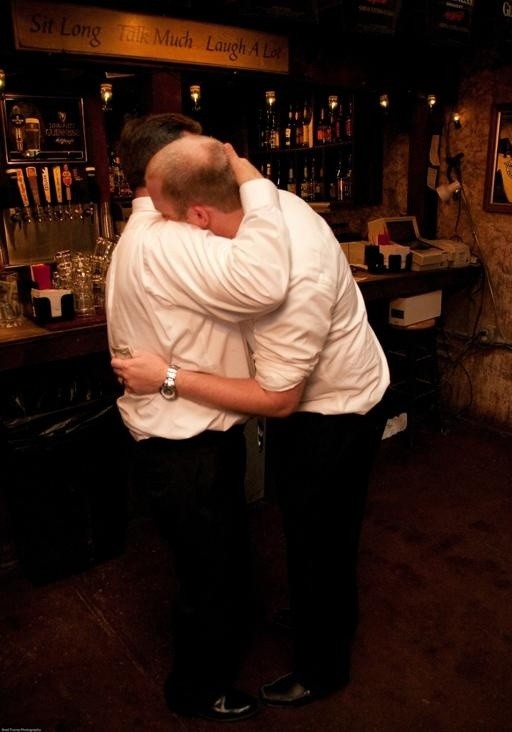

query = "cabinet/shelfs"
[{"left": 252, "top": 88, "right": 384, "bottom": 210}]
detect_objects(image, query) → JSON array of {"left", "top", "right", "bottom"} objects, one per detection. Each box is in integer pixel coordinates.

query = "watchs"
[{"left": 157, "top": 363, "right": 181, "bottom": 401}]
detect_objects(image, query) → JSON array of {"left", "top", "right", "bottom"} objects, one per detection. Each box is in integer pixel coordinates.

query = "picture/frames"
[{"left": 482, "top": 102, "right": 512, "bottom": 215}]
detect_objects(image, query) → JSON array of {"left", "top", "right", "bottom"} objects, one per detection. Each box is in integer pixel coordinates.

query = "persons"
[
  {"left": 108, "top": 133, "right": 395, "bottom": 708},
  {"left": 98, "top": 109, "right": 293, "bottom": 715}
]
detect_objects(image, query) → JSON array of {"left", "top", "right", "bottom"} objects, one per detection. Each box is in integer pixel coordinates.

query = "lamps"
[
  {"left": 436, "top": 180, "right": 464, "bottom": 244},
  {"left": 190, "top": 84, "right": 201, "bottom": 103},
  {"left": 328, "top": 93, "right": 340, "bottom": 109},
  {"left": 265, "top": 90, "right": 276, "bottom": 105},
  {"left": 452, "top": 112, "right": 461, "bottom": 129},
  {"left": 379, "top": 92, "right": 389, "bottom": 109},
  {"left": 429, "top": 93, "right": 438, "bottom": 106},
  {"left": 99, "top": 81, "right": 113, "bottom": 101}
]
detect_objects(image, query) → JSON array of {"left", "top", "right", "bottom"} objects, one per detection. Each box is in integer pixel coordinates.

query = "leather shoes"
[
  {"left": 191, "top": 679, "right": 260, "bottom": 723},
  {"left": 259, "top": 669, "right": 329, "bottom": 709}
]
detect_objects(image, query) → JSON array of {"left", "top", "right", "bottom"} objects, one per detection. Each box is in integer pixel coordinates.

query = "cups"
[
  {"left": 0, "top": 275, "right": 25, "bottom": 329},
  {"left": 52, "top": 237, "right": 114, "bottom": 319},
  {"left": 31, "top": 266, "right": 51, "bottom": 289}
]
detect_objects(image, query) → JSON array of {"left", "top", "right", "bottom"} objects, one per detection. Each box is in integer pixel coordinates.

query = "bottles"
[
  {"left": 285, "top": 102, "right": 356, "bottom": 145},
  {"left": 264, "top": 155, "right": 351, "bottom": 201}
]
